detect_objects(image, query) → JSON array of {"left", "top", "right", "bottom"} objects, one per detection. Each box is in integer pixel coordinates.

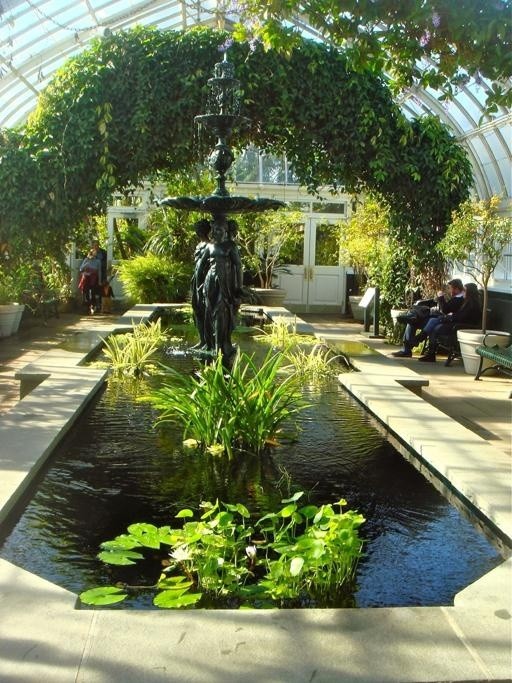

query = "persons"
[
  {"left": 198, "top": 215, "right": 246, "bottom": 374},
  {"left": 189, "top": 219, "right": 212, "bottom": 350},
  {"left": 81, "top": 238, "right": 108, "bottom": 309},
  {"left": 390, "top": 276, "right": 465, "bottom": 357},
  {"left": 97, "top": 279, "right": 115, "bottom": 313},
  {"left": 79, "top": 246, "right": 101, "bottom": 315},
  {"left": 226, "top": 219, "right": 243, "bottom": 319},
  {"left": 401, "top": 282, "right": 482, "bottom": 362}
]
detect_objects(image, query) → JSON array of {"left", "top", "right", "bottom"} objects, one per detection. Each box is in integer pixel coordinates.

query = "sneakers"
[{"left": 390, "top": 314, "right": 439, "bottom": 362}]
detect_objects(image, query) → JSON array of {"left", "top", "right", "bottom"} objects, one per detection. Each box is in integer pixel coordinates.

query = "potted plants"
[
  {"left": 0, "top": 265, "right": 30, "bottom": 337},
  {"left": 243, "top": 201, "right": 307, "bottom": 305},
  {"left": 333, "top": 204, "right": 412, "bottom": 327},
  {"left": 432, "top": 191, "right": 511, "bottom": 376}
]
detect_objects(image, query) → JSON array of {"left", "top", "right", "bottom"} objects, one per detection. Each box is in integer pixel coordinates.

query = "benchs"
[{"left": 421, "top": 308, "right": 512, "bottom": 400}]
